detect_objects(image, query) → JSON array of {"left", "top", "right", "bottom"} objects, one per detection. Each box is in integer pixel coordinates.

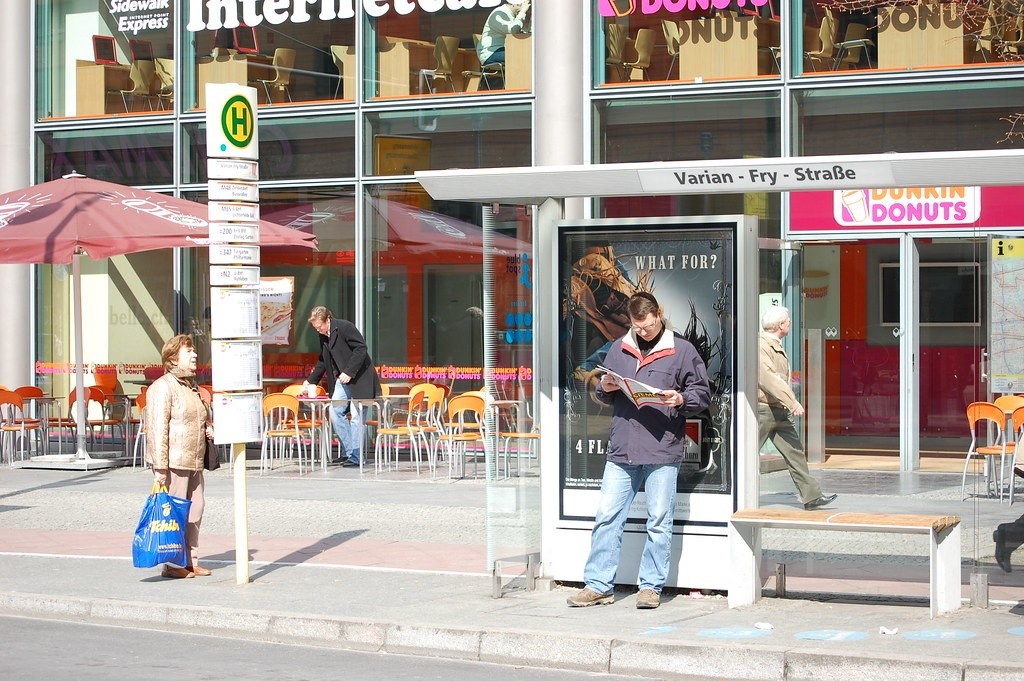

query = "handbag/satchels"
[
  {"left": 204, "top": 437, "right": 221, "bottom": 472},
  {"left": 132, "top": 482, "right": 192, "bottom": 568}
]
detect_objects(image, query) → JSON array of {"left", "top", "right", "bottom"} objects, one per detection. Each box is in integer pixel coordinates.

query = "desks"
[
  {"left": 77, "top": 60, "right": 162, "bottom": 115},
  {"left": 334, "top": 42, "right": 480, "bottom": 100},
  {"left": 196, "top": 53, "right": 285, "bottom": 107},
  {"left": 295, "top": 397, "right": 383, "bottom": 474},
  {"left": 606, "top": 23, "right": 631, "bottom": 82},
  {"left": 378, "top": 394, "right": 429, "bottom": 462},
  {"left": 504, "top": 33, "right": 533, "bottom": 91}
]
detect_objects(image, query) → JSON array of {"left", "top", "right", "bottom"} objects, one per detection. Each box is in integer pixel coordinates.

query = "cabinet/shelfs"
[
  {"left": 877, "top": 3, "right": 963, "bottom": 69},
  {"left": 679, "top": 17, "right": 822, "bottom": 77}
]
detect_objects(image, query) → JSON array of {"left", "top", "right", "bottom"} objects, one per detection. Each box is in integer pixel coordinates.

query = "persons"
[
  {"left": 757, "top": 306, "right": 838, "bottom": 512},
  {"left": 992, "top": 432, "right": 1024, "bottom": 573},
  {"left": 565, "top": 291, "right": 710, "bottom": 609},
  {"left": 146, "top": 335, "right": 214, "bottom": 580},
  {"left": 298, "top": 306, "right": 381, "bottom": 466},
  {"left": 475, "top": 0, "right": 531, "bottom": 65}
]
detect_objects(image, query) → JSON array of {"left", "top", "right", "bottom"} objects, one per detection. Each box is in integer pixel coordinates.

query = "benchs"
[{"left": 727, "top": 509, "right": 962, "bottom": 621}]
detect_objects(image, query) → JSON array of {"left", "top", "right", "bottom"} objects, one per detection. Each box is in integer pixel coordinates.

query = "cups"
[{"left": 308, "top": 384, "right": 316, "bottom": 398}]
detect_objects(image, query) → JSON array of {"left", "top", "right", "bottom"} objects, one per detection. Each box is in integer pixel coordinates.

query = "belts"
[{"left": 758, "top": 400, "right": 787, "bottom": 410}]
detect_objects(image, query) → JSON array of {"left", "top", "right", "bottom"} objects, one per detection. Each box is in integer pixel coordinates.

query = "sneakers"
[
  {"left": 567, "top": 586, "right": 614, "bottom": 607},
  {"left": 637, "top": 587, "right": 661, "bottom": 608}
]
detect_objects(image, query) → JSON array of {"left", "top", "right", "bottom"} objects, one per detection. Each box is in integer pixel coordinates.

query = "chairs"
[
  {"left": 661, "top": 19, "right": 680, "bottom": 82},
  {"left": 994, "top": 4, "right": 1024, "bottom": 63},
  {"left": 418, "top": 35, "right": 461, "bottom": 95},
  {"left": 154, "top": 57, "right": 174, "bottom": 112},
  {"left": 197, "top": 384, "right": 233, "bottom": 475},
  {"left": 768, "top": 12, "right": 807, "bottom": 75},
  {"left": 331, "top": 44, "right": 348, "bottom": 101},
  {"left": 805, "top": 16, "right": 839, "bottom": 73},
  {"left": 365, "top": 382, "right": 540, "bottom": 481},
  {"left": 828, "top": 22, "right": 868, "bottom": 72},
  {"left": 826, "top": 8, "right": 874, "bottom": 70},
  {"left": 473, "top": 33, "right": 506, "bottom": 90},
  {"left": 963, "top": 0, "right": 1001, "bottom": 65},
  {"left": 258, "top": 384, "right": 341, "bottom": 477},
  {"left": 256, "top": 47, "right": 297, "bottom": 104},
  {"left": 617, "top": 28, "right": 657, "bottom": 82},
  {"left": 960, "top": 394, "right": 1024, "bottom": 507},
  {"left": 0, "top": 366, "right": 164, "bottom": 471},
  {"left": 106, "top": 59, "right": 156, "bottom": 113}
]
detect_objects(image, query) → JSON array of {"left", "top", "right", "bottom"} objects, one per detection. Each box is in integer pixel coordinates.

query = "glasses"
[{"left": 629, "top": 316, "right": 658, "bottom": 332}]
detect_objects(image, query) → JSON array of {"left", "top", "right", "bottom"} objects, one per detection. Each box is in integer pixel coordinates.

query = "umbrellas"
[
  {"left": 0, "top": 169, "right": 320, "bottom": 459},
  {"left": 263, "top": 189, "right": 531, "bottom": 266}
]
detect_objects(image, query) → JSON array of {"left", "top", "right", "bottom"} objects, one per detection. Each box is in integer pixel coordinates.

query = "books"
[{"left": 595, "top": 364, "right": 668, "bottom": 410}]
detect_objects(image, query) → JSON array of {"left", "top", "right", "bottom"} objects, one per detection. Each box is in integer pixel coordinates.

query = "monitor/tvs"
[
  {"left": 131, "top": 40, "right": 153, "bottom": 63},
  {"left": 92, "top": 34, "right": 117, "bottom": 65},
  {"left": 211, "top": 23, "right": 259, "bottom": 54}
]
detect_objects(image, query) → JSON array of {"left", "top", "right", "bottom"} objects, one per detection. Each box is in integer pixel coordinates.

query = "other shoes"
[
  {"left": 186, "top": 565, "right": 212, "bottom": 577},
  {"left": 344, "top": 459, "right": 359, "bottom": 468},
  {"left": 161, "top": 563, "right": 194, "bottom": 578},
  {"left": 332, "top": 455, "right": 347, "bottom": 464}
]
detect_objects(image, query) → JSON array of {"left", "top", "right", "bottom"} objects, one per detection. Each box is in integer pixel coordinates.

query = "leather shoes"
[
  {"left": 804, "top": 492, "right": 837, "bottom": 511},
  {"left": 993, "top": 524, "right": 1012, "bottom": 572}
]
